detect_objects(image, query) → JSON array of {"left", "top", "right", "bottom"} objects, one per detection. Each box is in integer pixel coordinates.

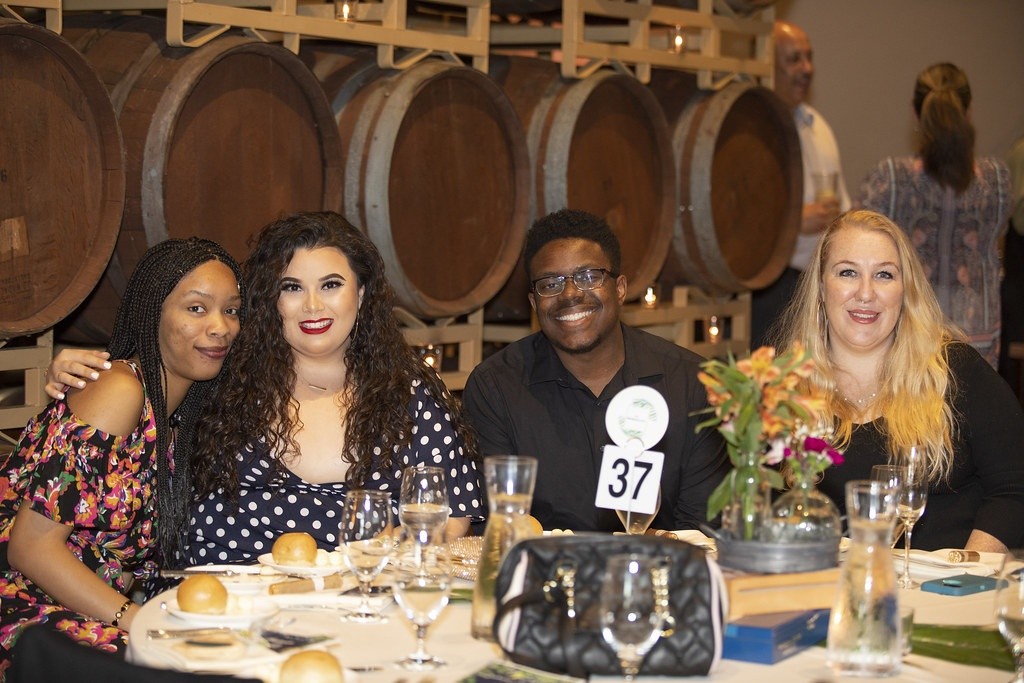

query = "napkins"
[{"left": 894, "top": 547, "right": 1006, "bottom": 584}]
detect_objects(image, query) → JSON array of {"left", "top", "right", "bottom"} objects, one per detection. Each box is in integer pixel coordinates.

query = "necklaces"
[
  {"left": 831, "top": 389, "right": 878, "bottom": 405},
  {"left": 289, "top": 364, "right": 330, "bottom": 392}
]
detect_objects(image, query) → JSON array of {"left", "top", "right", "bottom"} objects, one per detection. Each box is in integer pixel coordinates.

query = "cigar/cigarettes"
[
  {"left": 269, "top": 574, "right": 342, "bottom": 595},
  {"left": 645, "top": 529, "right": 677, "bottom": 541},
  {"left": 948, "top": 551, "right": 980, "bottom": 562}
]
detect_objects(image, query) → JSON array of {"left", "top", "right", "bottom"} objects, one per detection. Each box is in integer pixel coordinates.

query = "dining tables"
[{"left": 124, "top": 532, "right": 1024, "bottom": 683}]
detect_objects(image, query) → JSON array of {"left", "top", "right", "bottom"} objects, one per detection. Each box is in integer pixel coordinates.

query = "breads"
[
  {"left": 176, "top": 575, "right": 228, "bottom": 614},
  {"left": 279, "top": 649, "right": 344, "bottom": 683},
  {"left": 271, "top": 532, "right": 318, "bottom": 567},
  {"left": 526, "top": 515, "right": 544, "bottom": 537}
]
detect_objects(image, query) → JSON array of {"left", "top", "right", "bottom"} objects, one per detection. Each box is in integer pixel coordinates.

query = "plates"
[
  {"left": 257, "top": 550, "right": 342, "bottom": 577},
  {"left": 161, "top": 595, "right": 278, "bottom": 624},
  {"left": 353, "top": 539, "right": 397, "bottom": 556},
  {"left": 185, "top": 564, "right": 261, "bottom": 578}
]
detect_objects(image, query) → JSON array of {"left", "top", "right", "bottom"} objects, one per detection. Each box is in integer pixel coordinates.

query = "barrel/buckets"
[{"left": 0, "top": 13, "right": 806, "bottom": 345}]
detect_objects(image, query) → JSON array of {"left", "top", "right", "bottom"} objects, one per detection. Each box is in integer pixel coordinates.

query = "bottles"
[
  {"left": 473, "top": 457, "right": 541, "bottom": 639},
  {"left": 826, "top": 480, "right": 903, "bottom": 677}
]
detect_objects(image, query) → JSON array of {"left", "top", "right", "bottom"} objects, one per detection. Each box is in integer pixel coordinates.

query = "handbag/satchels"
[{"left": 494, "top": 535, "right": 725, "bottom": 682}]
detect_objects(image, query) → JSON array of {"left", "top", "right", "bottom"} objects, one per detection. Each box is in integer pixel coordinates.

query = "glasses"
[{"left": 531, "top": 267, "right": 619, "bottom": 298}]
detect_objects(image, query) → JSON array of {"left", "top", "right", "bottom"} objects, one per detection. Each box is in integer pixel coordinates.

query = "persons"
[
  {"left": 0, "top": 238, "right": 267, "bottom": 683},
  {"left": 459, "top": 210, "right": 736, "bottom": 534},
  {"left": 44, "top": 209, "right": 490, "bottom": 607},
  {"left": 770, "top": 206, "right": 1024, "bottom": 560},
  {"left": 747, "top": 22, "right": 852, "bottom": 355},
  {"left": 853, "top": 62, "right": 1012, "bottom": 372}
]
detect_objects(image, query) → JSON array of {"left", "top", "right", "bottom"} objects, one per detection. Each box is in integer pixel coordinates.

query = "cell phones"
[{"left": 921, "top": 573, "right": 1008, "bottom": 596}]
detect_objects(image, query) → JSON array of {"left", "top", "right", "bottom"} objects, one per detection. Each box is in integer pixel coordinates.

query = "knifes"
[{"left": 160, "top": 569, "right": 292, "bottom": 577}]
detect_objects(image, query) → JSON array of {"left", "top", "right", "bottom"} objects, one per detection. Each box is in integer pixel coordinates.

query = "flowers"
[{"left": 686, "top": 337, "right": 847, "bottom": 545}]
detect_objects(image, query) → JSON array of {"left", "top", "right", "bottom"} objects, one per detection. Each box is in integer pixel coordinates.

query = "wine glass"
[
  {"left": 338, "top": 466, "right": 450, "bottom": 673},
  {"left": 993, "top": 549, "right": 1024, "bottom": 683},
  {"left": 897, "top": 444, "right": 929, "bottom": 587}
]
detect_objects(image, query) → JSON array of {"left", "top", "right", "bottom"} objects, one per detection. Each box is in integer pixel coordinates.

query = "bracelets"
[{"left": 112, "top": 599, "right": 135, "bottom": 628}]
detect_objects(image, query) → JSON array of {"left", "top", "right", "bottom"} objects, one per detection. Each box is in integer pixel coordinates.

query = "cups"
[
  {"left": 640, "top": 281, "right": 661, "bottom": 311},
  {"left": 701, "top": 314, "right": 723, "bottom": 345},
  {"left": 613, "top": 484, "right": 661, "bottom": 535},
  {"left": 871, "top": 465, "right": 904, "bottom": 550}
]
